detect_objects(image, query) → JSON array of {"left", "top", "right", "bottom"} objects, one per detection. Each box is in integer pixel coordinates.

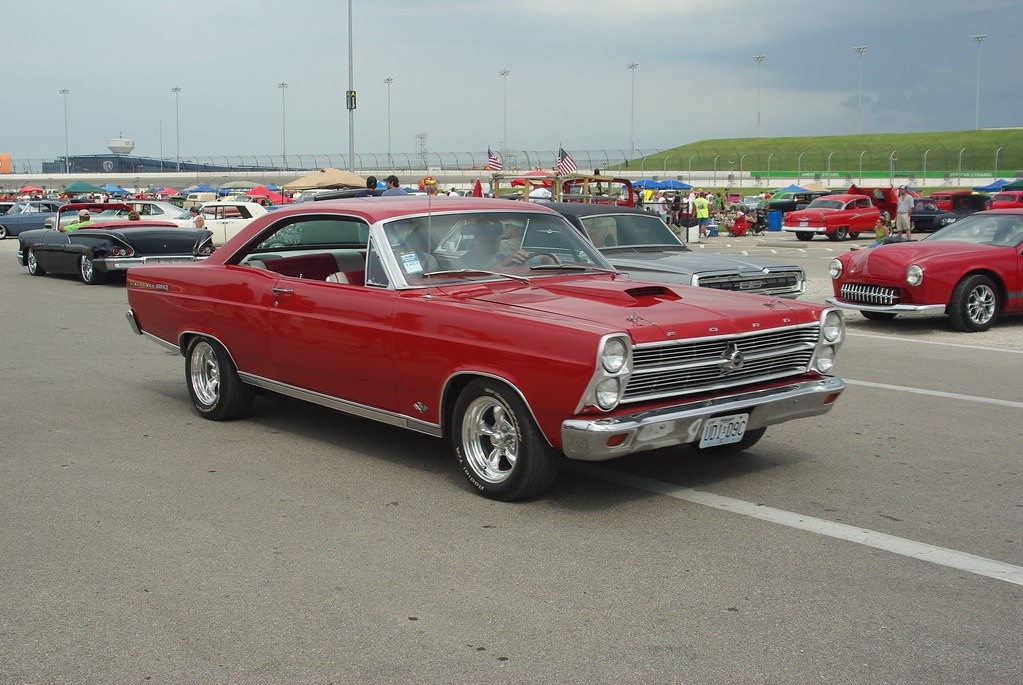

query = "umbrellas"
[{"left": 511, "top": 168, "right": 555, "bottom": 189}]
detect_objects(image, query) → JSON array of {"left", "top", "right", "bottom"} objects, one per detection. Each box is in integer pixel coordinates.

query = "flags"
[
  {"left": 557, "top": 146, "right": 577, "bottom": 177},
  {"left": 488, "top": 146, "right": 503, "bottom": 171}
]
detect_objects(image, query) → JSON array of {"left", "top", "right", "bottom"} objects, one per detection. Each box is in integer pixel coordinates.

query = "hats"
[
  {"left": 193, "top": 217, "right": 203, "bottom": 223},
  {"left": 128, "top": 211, "right": 140, "bottom": 220},
  {"left": 78, "top": 209, "right": 90, "bottom": 216},
  {"left": 383, "top": 175, "right": 399, "bottom": 182},
  {"left": 898, "top": 185, "right": 908, "bottom": 190},
  {"left": 367, "top": 176, "right": 377, "bottom": 183}
]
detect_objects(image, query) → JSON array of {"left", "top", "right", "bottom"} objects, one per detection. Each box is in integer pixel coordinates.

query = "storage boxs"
[{"left": 704, "top": 225, "right": 719, "bottom": 237}]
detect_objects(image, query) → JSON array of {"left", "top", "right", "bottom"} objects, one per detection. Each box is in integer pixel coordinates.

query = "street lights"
[
  {"left": 279, "top": 83, "right": 288, "bottom": 170},
  {"left": 628, "top": 63, "right": 640, "bottom": 149},
  {"left": 171, "top": 86, "right": 181, "bottom": 171},
  {"left": 971, "top": 33, "right": 989, "bottom": 129},
  {"left": 385, "top": 78, "right": 394, "bottom": 160},
  {"left": 58, "top": 89, "right": 70, "bottom": 172},
  {"left": 853, "top": 46, "right": 869, "bottom": 134},
  {"left": 498, "top": 69, "right": 511, "bottom": 151},
  {"left": 753, "top": 56, "right": 766, "bottom": 136}
]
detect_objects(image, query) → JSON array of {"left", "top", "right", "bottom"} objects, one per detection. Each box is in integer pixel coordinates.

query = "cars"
[
  {"left": 436, "top": 201, "right": 809, "bottom": 301},
  {"left": 0, "top": 193, "right": 307, "bottom": 248},
  {"left": 16, "top": 204, "right": 215, "bottom": 284},
  {"left": 828, "top": 206, "right": 1023, "bottom": 332},
  {"left": 125, "top": 196, "right": 847, "bottom": 501},
  {"left": 659, "top": 190, "right": 680, "bottom": 200},
  {"left": 768, "top": 191, "right": 830, "bottom": 214},
  {"left": 781, "top": 184, "right": 898, "bottom": 240},
  {"left": 731, "top": 191, "right": 794, "bottom": 212},
  {"left": 907, "top": 188, "right": 1023, "bottom": 233}
]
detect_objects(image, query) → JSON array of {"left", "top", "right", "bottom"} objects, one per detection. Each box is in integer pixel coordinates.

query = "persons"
[
  {"left": 449, "top": 216, "right": 553, "bottom": 272},
  {"left": 58, "top": 209, "right": 94, "bottom": 233},
  {"left": 592, "top": 185, "right": 772, "bottom": 237},
  {"left": 127, "top": 211, "right": 141, "bottom": 222},
  {"left": 0, "top": 191, "right": 162, "bottom": 204},
  {"left": 851, "top": 217, "right": 889, "bottom": 252},
  {"left": 896, "top": 185, "right": 914, "bottom": 240},
  {"left": 354, "top": 176, "right": 473, "bottom": 197},
  {"left": 188, "top": 207, "right": 208, "bottom": 229}
]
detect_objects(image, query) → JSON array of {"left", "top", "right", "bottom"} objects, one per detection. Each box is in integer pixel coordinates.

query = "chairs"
[
  {"left": 727, "top": 213, "right": 767, "bottom": 236},
  {"left": 327, "top": 245, "right": 429, "bottom": 288}
]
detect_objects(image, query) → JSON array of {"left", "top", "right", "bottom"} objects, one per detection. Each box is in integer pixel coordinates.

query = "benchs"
[
  {"left": 243, "top": 250, "right": 440, "bottom": 281},
  {"left": 77, "top": 221, "right": 178, "bottom": 229}
]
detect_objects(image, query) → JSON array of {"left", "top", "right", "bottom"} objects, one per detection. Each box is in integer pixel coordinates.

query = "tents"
[
  {"left": 973, "top": 178, "right": 1023, "bottom": 191},
  {"left": 19, "top": 181, "right": 293, "bottom": 203},
  {"left": 770, "top": 183, "right": 832, "bottom": 193},
  {"left": 621, "top": 179, "right": 694, "bottom": 192},
  {"left": 282, "top": 168, "right": 369, "bottom": 204}
]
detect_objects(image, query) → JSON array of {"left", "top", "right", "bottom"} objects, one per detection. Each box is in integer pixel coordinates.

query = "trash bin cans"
[
  {"left": 767, "top": 209, "right": 782, "bottom": 231},
  {"left": 680, "top": 218, "right": 700, "bottom": 242}
]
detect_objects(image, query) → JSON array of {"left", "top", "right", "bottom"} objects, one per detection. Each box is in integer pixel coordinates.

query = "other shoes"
[
  {"left": 706, "top": 230, "right": 711, "bottom": 238},
  {"left": 698, "top": 234, "right": 703, "bottom": 238}
]
